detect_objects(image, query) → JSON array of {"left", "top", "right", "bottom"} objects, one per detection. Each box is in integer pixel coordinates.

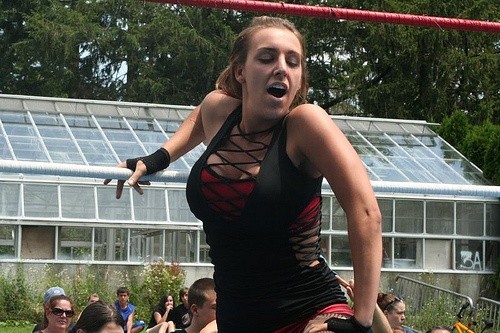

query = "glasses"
[
  {"left": 384, "top": 295, "right": 405, "bottom": 311},
  {"left": 51, "top": 308, "right": 74, "bottom": 317}
]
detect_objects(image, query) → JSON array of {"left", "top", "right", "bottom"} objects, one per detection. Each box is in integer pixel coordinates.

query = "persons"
[
  {"left": 174, "top": 287, "right": 190, "bottom": 329},
  {"left": 33, "top": 287, "right": 66, "bottom": 333},
  {"left": 35, "top": 296, "right": 73, "bottom": 333},
  {"left": 343, "top": 279, "right": 454, "bottom": 333},
  {"left": 113, "top": 287, "right": 145, "bottom": 333},
  {"left": 71, "top": 300, "right": 125, "bottom": 333},
  {"left": 146, "top": 294, "right": 175, "bottom": 333},
  {"left": 87, "top": 293, "right": 100, "bottom": 303},
  {"left": 171, "top": 278, "right": 217, "bottom": 333},
  {"left": 104, "top": 16, "right": 383, "bottom": 333}
]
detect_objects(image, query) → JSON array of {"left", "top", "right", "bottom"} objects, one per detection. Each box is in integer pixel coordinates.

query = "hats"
[{"left": 43, "top": 286, "right": 65, "bottom": 304}]
